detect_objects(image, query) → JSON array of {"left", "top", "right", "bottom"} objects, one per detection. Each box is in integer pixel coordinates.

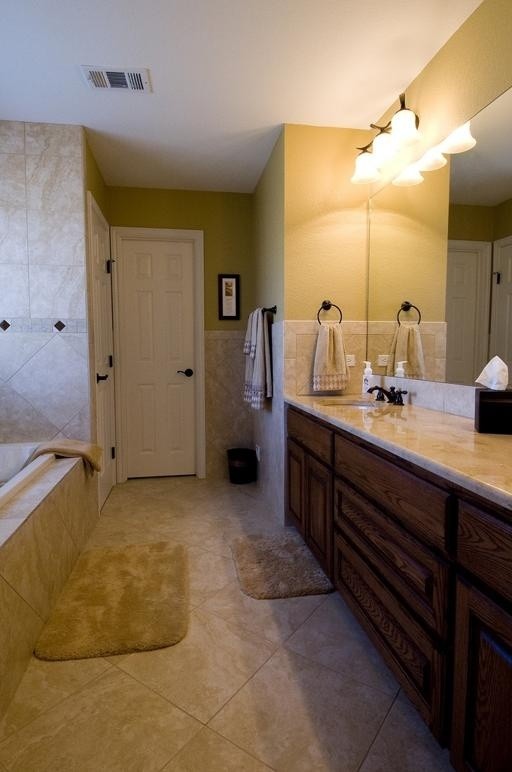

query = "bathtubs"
[{"left": 0, "top": 442, "right": 55, "bottom": 517}]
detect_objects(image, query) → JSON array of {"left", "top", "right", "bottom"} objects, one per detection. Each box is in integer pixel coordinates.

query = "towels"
[
  {"left": 240, "top": 308, "right": 274, "bottom": 412},
  {"left": 311, "top": 325, "right": 352, "bottom": 394},
  {"left": 16, "top": 440, "right": 106, "bottom": 478},
  {"left": 389, "top": 323, "right": 427, "bottom": 377}
]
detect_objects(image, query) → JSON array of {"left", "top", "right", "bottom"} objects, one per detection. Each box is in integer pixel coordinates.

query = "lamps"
[{"left": 351, "top": 90, "right": 421, "bottom": 184}]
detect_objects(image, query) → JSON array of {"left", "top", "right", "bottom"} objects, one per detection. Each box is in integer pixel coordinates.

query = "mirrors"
[{"left": 365, "top": 88, "right": 512, "bottom": 387}]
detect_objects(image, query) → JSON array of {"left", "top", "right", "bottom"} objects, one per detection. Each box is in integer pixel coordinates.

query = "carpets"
[
  {"left": 34, "top": 543, "right": 188, "bottom": 663},
  {"left": 226, "top": 527, "right": 335, "bottom": 599}
]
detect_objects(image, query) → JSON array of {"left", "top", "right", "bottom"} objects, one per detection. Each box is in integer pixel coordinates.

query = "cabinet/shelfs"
[
  {"left": 283, "top": 406, "right": 335, "bottom": 583},
  {"left": 329, "top": 435, "right": 451, "bottom": 749},
  {"left": 448, "top": 498, "right": 511, "bottom": 772}
]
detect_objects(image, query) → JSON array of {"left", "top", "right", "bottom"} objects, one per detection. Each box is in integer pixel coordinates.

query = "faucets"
[{"left": 367, "top": 386, "right": 396, "bottom": 402}]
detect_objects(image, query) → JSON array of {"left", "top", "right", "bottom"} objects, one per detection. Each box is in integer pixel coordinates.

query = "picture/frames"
[{"left": 215, "top": 271, "right": 241, "bottom": 321}]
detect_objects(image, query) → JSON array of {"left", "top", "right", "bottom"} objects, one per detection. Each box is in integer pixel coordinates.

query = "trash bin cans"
[{"left": 227, "top": 447, "right": 258, "bottom": 485}]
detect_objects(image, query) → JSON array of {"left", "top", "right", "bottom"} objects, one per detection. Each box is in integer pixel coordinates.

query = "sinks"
[{"left": 320, "top": 399, "right": 384, "bottom": 407}]
